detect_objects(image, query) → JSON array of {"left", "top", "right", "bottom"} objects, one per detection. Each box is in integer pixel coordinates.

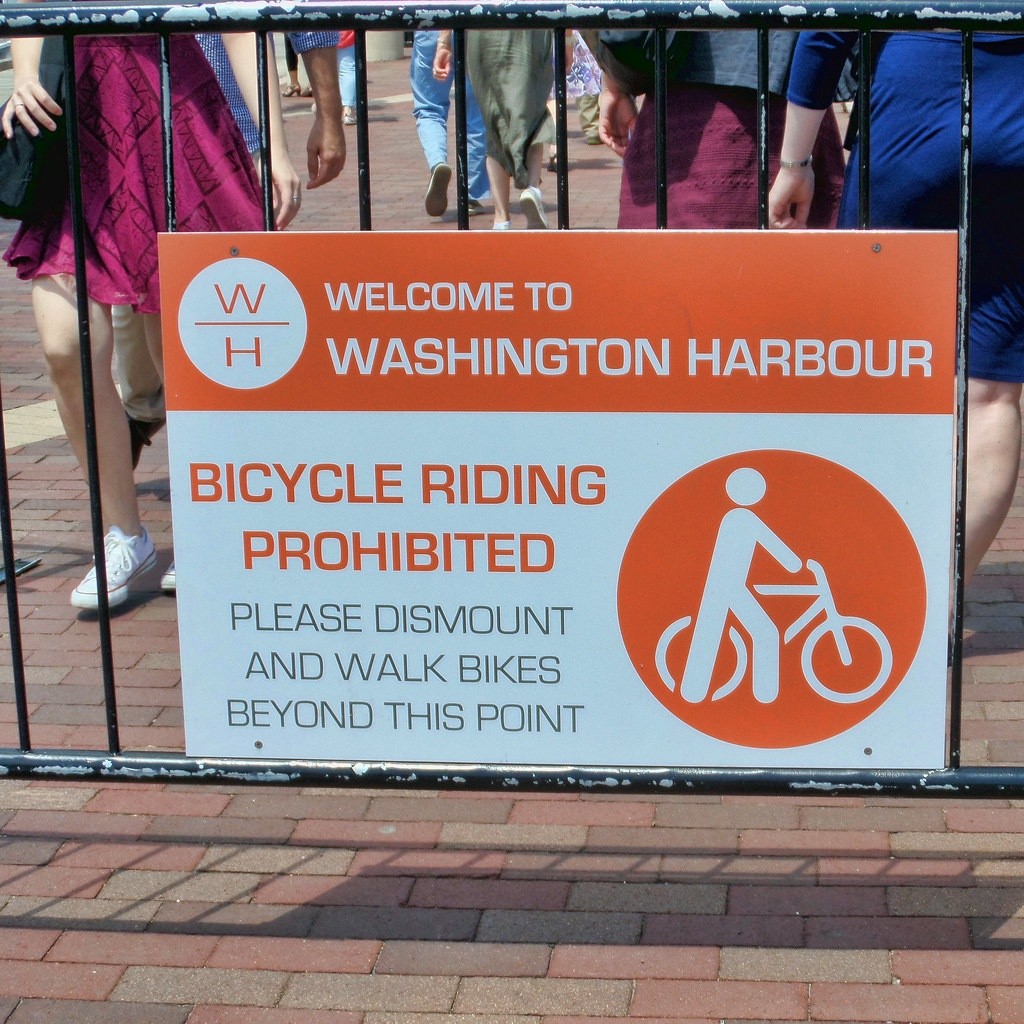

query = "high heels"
[
  {"left": 547, "top": 153, "right": 558, "bottom": 172},
  {"left": 282, "top": 84, "right": 301, "bottom": 97},
  {"left": 301, "top": 86, "right": 313, "bottom": 97}
]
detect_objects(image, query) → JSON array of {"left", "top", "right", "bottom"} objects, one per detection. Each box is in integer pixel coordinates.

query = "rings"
[
  {"left": 432, "top": 68, "right": 435, "bottom": 72},
  {"left": 292, "top": 195, "right": 298, "bottom": 199}
]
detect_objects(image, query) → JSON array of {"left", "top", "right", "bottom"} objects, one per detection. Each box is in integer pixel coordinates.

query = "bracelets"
[{"left": 780, "top": 155, "right": 813, "bottom": 168}]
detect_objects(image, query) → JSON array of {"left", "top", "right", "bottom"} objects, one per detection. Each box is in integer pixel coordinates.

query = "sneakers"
[
  {"left": 70, "top": 525, "right": 157, "bottom": 610},
  {"left": 160, "top": 559, "right": 176, "bottom": 591}
]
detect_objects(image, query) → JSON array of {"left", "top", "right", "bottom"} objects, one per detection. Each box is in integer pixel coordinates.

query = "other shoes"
[
  {"left": 425, "top": 163, "right": 452, "bottom": 215},
  {"left": 467, "top": 198, "right": 484, "bottom": 216},
  {"left": 125, "top": 414, "right": 166, "bottom": 469},
  {"left": 586, "top": 136, "right": 604, "bottom": 144},
  {"left": 520, "top": 188, "right": 549, "bottom": 228},
  {"left": 344, "top": 112, "right": 357, "bottom": 125}
]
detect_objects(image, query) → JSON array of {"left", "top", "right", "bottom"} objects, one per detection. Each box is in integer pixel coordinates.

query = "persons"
[
  {"left": 408, "top": 30, "right": 495, "bottom": 216},
  {"left": 281, "top": 30, "right": 314, "bottom": 98},
  {"left": 597, "top": 30, "right": 865, "bottom": 233},
  {"left": 89, "top": 0, "right": 349, "bottom": 471},
  {"left": 767, "top": 30, "right": 1023, "bottom": 647},
  {"left": 431, "top": 27, "right": 573, "bottom": 231},
  {"left": 311, "top": 30, "right": 357, "bottom": 124},
  {"left": 0, "top": 32, "right": 302, "bottom": 610},
  {"left": 535, "top": 26, "right": 652, "bottom": 176}
]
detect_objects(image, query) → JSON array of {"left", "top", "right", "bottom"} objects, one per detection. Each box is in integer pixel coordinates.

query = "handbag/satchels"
[
  {"left": 596, "top": 28, "right": 698, "bottom": 96},
  {"left": 0, "top": 81, "right": 71, "bottom": 219}
]
